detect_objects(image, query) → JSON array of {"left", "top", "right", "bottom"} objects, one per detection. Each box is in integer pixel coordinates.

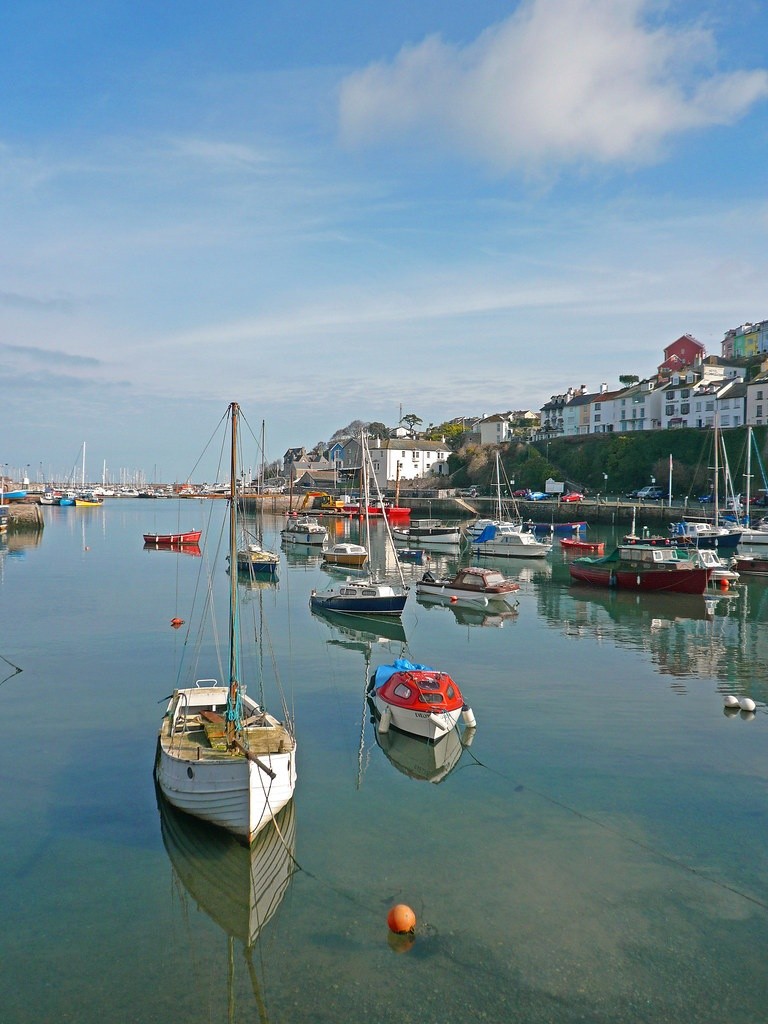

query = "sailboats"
[
  {"left": 155, "top": 403, "right": 298, "bottom": 851},
  {"left": 0, "top": 441, "right": 255, "bottom": 535},
  {"left": 159, "top": 789, "right": 295, "bottom": 1024}
]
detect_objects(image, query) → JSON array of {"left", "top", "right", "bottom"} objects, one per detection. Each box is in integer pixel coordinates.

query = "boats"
[
  {"left": 570, "top": 406, "right": 768, "bottom": 593},
  {"left": 225, "top": 570, "right": 280, "bottom": 603},
  {"left": 374, "top": 717, "right": 463, "bottom": 784},
  {"left": 368, "top": 659, "right": 465, "bottom": 743},
  {"left": 311, "top": 606, "right": 408, "bottom": 790},
  {"left": 280, "top": 517, "right": 328, "bottom": 544},
  {"left": 282, "top": 457, "right": 411, "bottom": 516},
  {"left": 143, "top": 528, "right": 202, "bottom": 545},
  {"left": 568, "top": 582, "right": 713, "bottom": 623},
  {"left": 226, "top": 528, "right": 281, "bottom": 573},
  {"left": 417, "top": 593, "right": 519, "bottom": 643},
  {"left": 310, "top": 428, "right": 410, "bottom": 616},
  {"left": 143, "top": 543, "right": 202, "bottom": 557},
  {"left": 391, "top": 450, "right": 605, "bottom": 556},
  {"left": 417, "top": 557, "right": 520, "bottom": 602},
  {"left": 320, "top": 543, "right": 367, "bottom": 564},
  {"left": 280, "top": 543, "right": 328, "bottom": 572}
]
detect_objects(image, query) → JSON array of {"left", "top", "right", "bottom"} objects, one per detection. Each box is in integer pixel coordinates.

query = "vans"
[{"left": 637, "top": 487, "right": 664, "bottom": 499}]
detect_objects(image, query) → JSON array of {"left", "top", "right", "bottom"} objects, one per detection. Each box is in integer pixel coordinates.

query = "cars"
[
  {"left": 512, "top": 490, "right": 550, "bottom": 501},
  {"left": 698, "top": 492, "right": 768, "bottom": 506},
  {"left": 626, "top": 490, "right": 639, "bottom": 497},
  {"left": 562, "top": 492, "right": 585, "bottom": 502}
]
